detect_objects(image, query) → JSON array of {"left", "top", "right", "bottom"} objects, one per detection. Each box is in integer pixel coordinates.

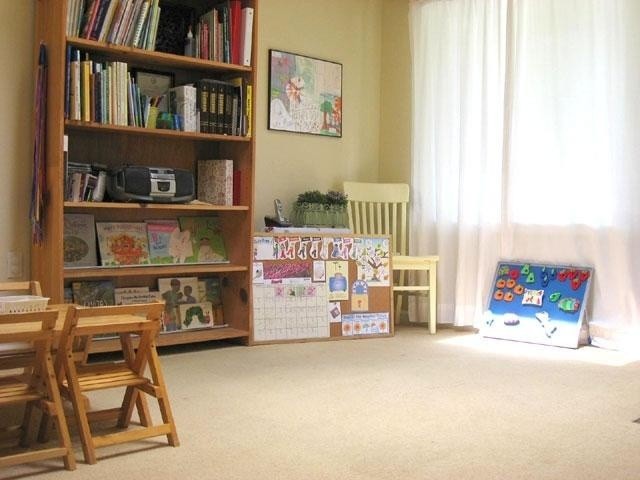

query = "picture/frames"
[{"left": 268, "top": 47, "right": 343, "bottom": 139}]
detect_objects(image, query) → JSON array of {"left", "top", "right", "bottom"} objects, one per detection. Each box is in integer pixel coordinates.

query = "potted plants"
[{"left": 292, "top": 189, "right": 349, "bottom": 229}]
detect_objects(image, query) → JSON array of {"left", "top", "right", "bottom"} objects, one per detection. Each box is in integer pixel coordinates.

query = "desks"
[{"left": 1, "top": 302, "right": 156, "bottom": 395}]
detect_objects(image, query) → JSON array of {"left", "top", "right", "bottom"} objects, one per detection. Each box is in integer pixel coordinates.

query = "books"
[
  {"left": 62, "top": 43, "right": 143, "bottom": 128},
  {"left": 61, "top": 212, "right": 97, "bottom": 268},
  {"left": 178, "top": 216, "right": 228, "bottom": 264},
  {"left": 95, "top": 222, "right": 151, "bottom": 266},
  {"left": 65, "top": 0, "right": 161, "bottom": 52},
  {"left": 168, "top": 78, "right": 252, "bottom": 138},
  {"left": 145, "top": 218, "right": 186, "bottom": 266},
  {"left": 194, "top": 1, "right": 254, "bottom": 66},
  {"left": 64, "top": 276, "right": 227, "bottom": 340}
]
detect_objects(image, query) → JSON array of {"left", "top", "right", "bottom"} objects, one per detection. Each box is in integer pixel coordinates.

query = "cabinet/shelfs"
[{"left": 33, "top": 1, "right": 255, "bottom": 351}]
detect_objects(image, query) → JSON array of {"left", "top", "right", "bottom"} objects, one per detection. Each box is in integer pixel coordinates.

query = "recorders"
[{"left": 108, "top": 165, "right": 196, "bottom": 204}]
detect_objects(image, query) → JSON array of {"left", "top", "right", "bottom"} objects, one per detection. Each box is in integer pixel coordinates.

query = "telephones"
[{"left": 265, "top": 199, "right": 292, "bottom": 226}]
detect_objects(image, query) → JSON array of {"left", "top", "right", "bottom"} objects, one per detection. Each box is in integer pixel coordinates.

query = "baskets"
[{"left": 289, "top": 202, "right": 350, "bottom": 228}]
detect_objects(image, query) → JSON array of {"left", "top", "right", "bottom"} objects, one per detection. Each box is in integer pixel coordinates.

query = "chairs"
[
  {"left": 342, "top": 182, "right": 440, "bottom": 334},
  {"left": 41, "top": 303, "right": 182, "bottom": 464},
  {"left": 1, "top": 280, "right": 43, "bottom": 298},
  {"left": 0, "top": 311, "right": 77, "bottom": 473}
]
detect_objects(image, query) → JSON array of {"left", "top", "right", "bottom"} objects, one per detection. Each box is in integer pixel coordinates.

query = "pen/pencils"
[{"left": 149, "top": 90, "right": 166, "bottom": 107}]
